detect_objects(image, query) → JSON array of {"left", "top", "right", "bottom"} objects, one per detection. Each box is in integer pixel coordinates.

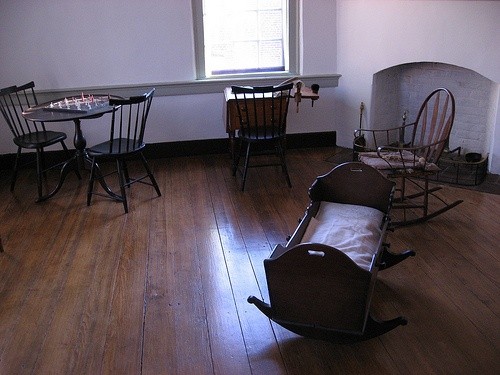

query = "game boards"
[{"left": 40, "top": 92, "right": 114, "bottom": 114}]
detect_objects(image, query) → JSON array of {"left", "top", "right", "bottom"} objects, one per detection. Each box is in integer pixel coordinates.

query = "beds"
[{"left": 248, "top": 162, "right": 417, "bottom": 344}]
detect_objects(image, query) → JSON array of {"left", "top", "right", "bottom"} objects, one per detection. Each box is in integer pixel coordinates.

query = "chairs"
[
  {"left": 0, "top": 81, "right": 82, "bottom": 203},
  {"left": 353, "top": 88, "right": 464, "bottom": 228},
  {"left": 231, "top": 83, "right": 295, "bottom": 191},
  {"left": 87, "top": 87, "right": 161, "bottom": 214}
]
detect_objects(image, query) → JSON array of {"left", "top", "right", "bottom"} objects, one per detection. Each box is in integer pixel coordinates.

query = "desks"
[
  {"left": 22, "top": 94, "right": 123, "bottom": 203},
  {"left": 223, "top": 86, "right": 320, "bottom": 170}
]
